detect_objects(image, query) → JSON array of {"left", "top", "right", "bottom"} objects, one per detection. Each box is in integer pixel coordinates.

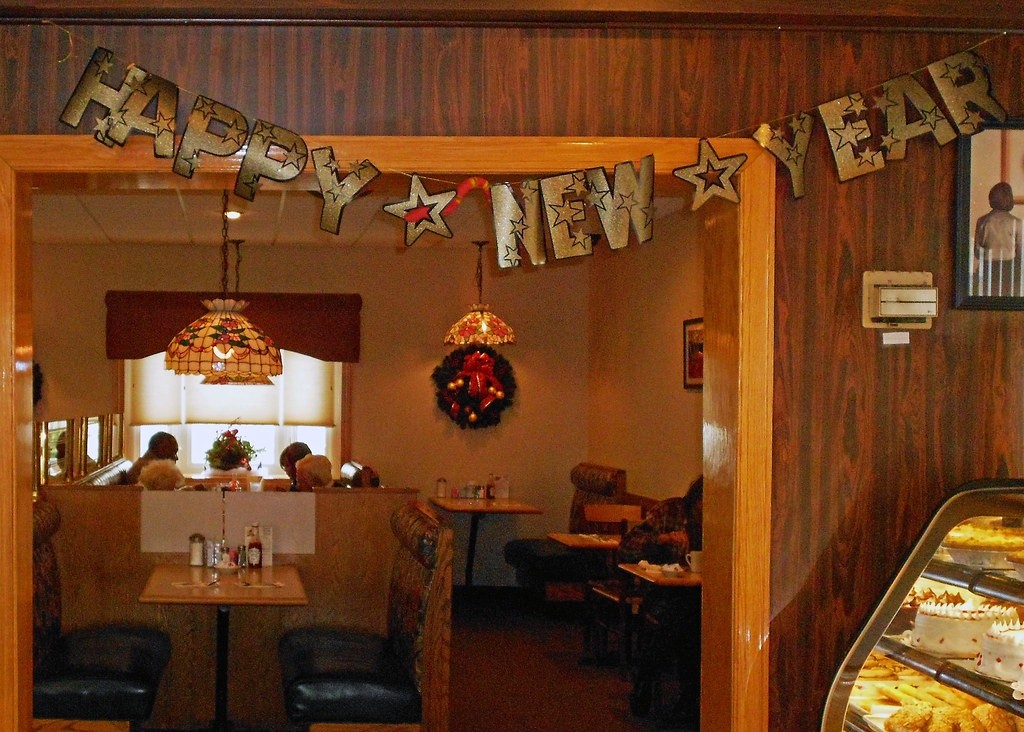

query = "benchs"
[
  {"left": 34, "top": 498, "right": 173, "bottom": 732},
  {"left": 504, "top": 461, "right": 626, "bottom": 614},
  {"left": 333, "top": 458, "right": 369, "bottom": 489},
  {"left": 352, "top": 460, "right": 381, "bottom": 488},
  {"left": 71, "top": 458, "right": 135, "bottom": 486},
  {"left": 278, "top": 500, "right": 452, "bottom": 732}
]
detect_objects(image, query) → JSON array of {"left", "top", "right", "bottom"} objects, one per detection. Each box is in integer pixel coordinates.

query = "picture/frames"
[
  {"left": 952, "top": 114, "right": 1024, "bottom": 312},
  {"left": 683, "top": 317, "right": 704, "bottom": 389}
]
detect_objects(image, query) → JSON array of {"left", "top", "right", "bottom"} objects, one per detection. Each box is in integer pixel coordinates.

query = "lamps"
[
  {"left": 444, "top": 237, "right": 519, "bottom": 349},
  {"left": 200, "top": 236, "right": 279, "bottom": 387},
  {"left": 166, "top": 191, "right": 283, "bottom": 378}
]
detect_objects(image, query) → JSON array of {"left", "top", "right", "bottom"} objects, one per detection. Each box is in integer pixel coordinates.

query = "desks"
[
  {"left": 546, "top": 532, "right": 628, "bottom": 550},
  {"left": 139, "top": 561, "right": 308, "bottom": 732},
  {"left": 431, "top": 497, "right": 545, "bottom": 605}
]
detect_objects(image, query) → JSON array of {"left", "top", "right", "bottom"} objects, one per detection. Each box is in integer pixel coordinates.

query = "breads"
[
  {"left": 861, "top": 648, "right": 926, "bottom": 681},
  {"left": 859, "top": 681, "right": 1024, "bottom": 732}
]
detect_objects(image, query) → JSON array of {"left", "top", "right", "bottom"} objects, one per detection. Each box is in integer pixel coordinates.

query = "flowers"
[
  {"left": 432, "top": 345, "right": 518, "bottom": 429},
  {"left": 204, "top": 419, "right": 258, "bottom": 472}
]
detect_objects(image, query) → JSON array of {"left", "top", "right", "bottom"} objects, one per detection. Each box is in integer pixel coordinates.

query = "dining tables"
[{"left": 617, "top": 560, "right": 702, "bottom": 589}]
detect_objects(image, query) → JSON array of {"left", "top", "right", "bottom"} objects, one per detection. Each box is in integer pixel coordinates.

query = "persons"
[
  {"left": 974, "top": 182, "right": 1022, "bottom": 293},
  {"left": 616, "top": 476, "right": 703, "bottom": 718},
  {"left": 140, "top": 459, "right": 183, "bottom": 490},
  {"left": 295, "top": 454, "right": 347, "bottom": 492},
  {"left": 56, "top": 430, "right": 95, "bottom": 468},
  {"left": 125, "top": 432, "right": 180, "bottom": 485},
  {"left": 273, "top": 442, "right": 313, "bottom": 492}
]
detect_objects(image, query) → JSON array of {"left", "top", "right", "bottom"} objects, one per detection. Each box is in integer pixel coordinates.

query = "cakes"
[{"left": 911, "top": 528, "right": 1024, "bottom": 684}]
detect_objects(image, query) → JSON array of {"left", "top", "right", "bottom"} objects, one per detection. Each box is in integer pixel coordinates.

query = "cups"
[{"left": 685, "top": 550, "right": 702, "bottom": 573}]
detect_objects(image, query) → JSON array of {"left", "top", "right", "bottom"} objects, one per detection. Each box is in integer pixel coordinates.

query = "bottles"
[
  {"left": 187, "top": 520, "right": 264, "bottom": 571},
  {"left": 436, "top": 472, "right": 496, "bottom": 504}
]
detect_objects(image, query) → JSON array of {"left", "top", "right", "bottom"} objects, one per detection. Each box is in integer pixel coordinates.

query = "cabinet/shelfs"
[{"left": 820, "top": 478, "right": 1023, "bottom": 731}]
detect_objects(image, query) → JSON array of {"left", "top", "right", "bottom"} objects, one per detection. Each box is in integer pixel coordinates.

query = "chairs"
[
  {"left": 587, "top": 583, "right": 646, "bottom": 678},
  {"left": 584, "top": 503, "right": 644, "bottom": 538}
]
